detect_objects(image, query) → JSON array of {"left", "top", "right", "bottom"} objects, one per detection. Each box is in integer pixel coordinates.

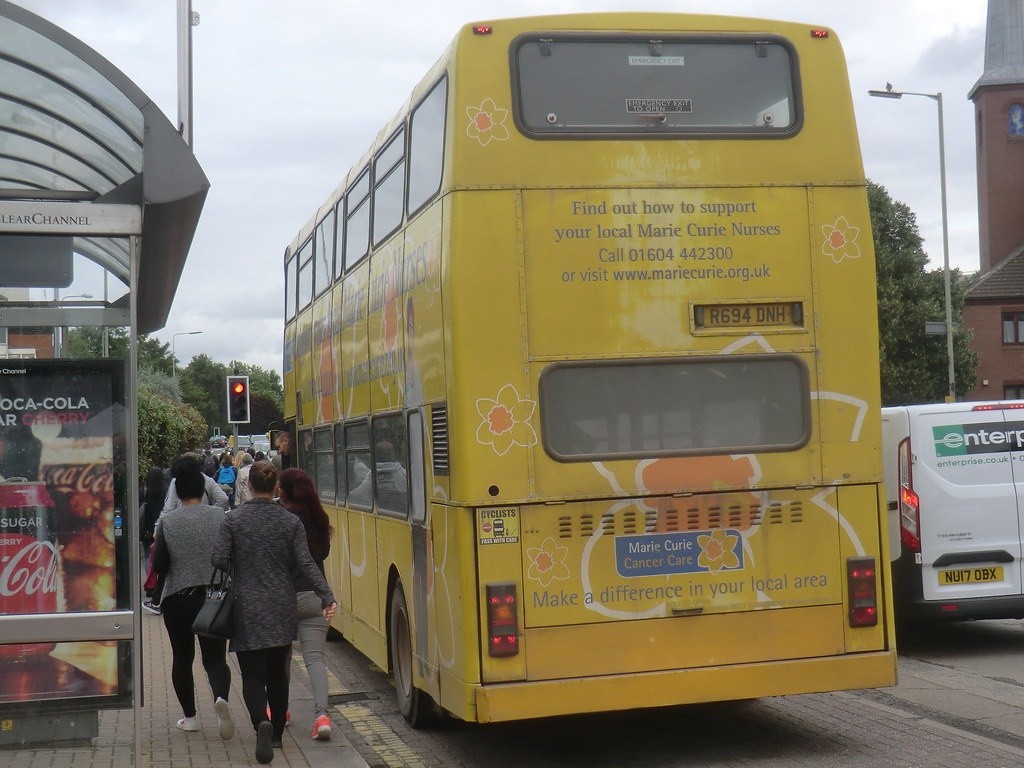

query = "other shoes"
[
  {"left": 255, "top": 721, "right": 273, "bottom": 764},
  {"left": 272, "top": 736, "right": 281, "bottom": 748}
]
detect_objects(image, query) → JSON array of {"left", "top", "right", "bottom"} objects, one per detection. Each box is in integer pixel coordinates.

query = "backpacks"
[
  {"left": 377, "top": 465, "right": 403, "bottom": 510},
  {"left": 217, "top": 465, "right": 236, "bottom": 486}
]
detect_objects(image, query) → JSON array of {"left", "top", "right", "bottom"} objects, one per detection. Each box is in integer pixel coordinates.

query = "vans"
[{"left": 881, "top": 399, "right": 1024, "bottom": 620}]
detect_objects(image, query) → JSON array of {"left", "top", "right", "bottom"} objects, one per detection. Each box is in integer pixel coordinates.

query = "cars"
[{"left": 210, "top": 434, "right": 270, "bottom": 456}]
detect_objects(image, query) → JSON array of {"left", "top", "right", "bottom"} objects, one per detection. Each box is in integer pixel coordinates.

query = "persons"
[
  {"left": 152, "top": 471, "right": 244, "bottom": 740},
  {"left": 348, "top": 441, "right": 408, "bottom": 513},
  {"left": 134, "top": 442, "right": 307, "bottom": 616},
  {"left": 264, "top": 467, "right": 336, "bottom": 739},
  {"left": 272, "top": 429, "right": 291, "bottom": 458},
  {"left": 209, "top": 459, "right": 339, "bottom": 765}
]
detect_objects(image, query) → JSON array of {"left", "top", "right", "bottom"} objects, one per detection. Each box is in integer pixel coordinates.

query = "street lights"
[
  {"left": 868, "top": 89, "right": 956, "bottom": 402},
  {"left": 172, "top": 331, "right": 203, "bottom": 381}
]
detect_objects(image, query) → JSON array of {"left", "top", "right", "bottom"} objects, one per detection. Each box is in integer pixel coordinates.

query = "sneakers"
[
  {"left": 265, "top": 707, "right": 290, "bottom": 726},
  {"left": 176, "top": 716, "right": 199, "bottom": 732},
  {"left": 312, "top": 716, "right": 331, "bottom": 740},
  {"left": 142, "top": 601, "right": 163, "bottom": 614},
  {"left": 215, "top": 696, "right": 234, "bottom": 740}
]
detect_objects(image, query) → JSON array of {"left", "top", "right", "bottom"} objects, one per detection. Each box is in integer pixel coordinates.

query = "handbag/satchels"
[
  {"left": 191, "top": 560, "right": 234, "bottom": 640},
  {"left": 140, "top": 500, "right": 154, "bottom": 546}
]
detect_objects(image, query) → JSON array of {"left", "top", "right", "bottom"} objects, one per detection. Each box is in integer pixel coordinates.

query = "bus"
[{"left": 265, "top": 10, "right": 898, "bottom": 730}]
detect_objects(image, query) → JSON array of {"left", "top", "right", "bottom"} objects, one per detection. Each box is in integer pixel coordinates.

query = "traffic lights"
[{"left": 226, "top": 376, "right": 250, "bottom": 424}]
